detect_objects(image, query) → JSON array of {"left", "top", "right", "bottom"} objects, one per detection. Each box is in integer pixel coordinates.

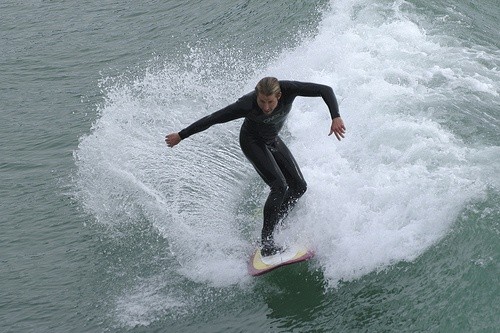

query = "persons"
[{"left": 166, "top": 77, "right": 346, "bottom": 257}]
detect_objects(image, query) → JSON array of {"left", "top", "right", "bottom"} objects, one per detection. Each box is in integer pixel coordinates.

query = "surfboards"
[{"left": 247, "top": 244, "right": 315, "bottom": 276}]
